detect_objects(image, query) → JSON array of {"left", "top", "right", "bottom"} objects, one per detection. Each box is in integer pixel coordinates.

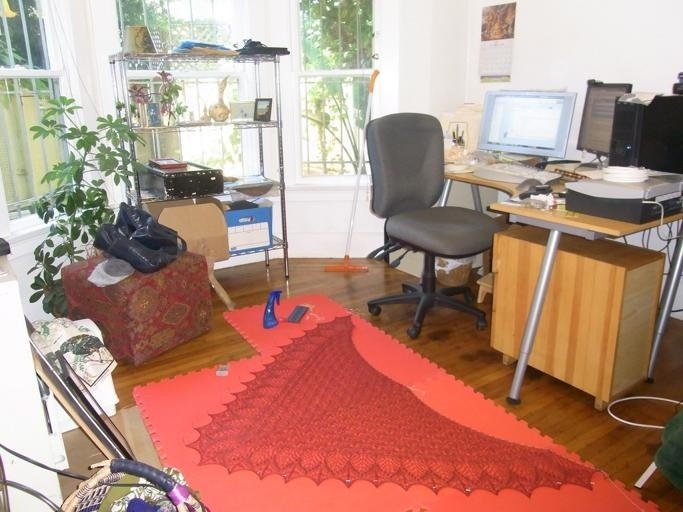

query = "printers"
[{"left": 564, "top": 165, "right": 682, "bottom": 224}]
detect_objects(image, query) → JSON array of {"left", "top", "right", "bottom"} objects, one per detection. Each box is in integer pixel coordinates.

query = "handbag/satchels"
[{"left": 93, "top": 202, "right": 186, "bottom": 272}]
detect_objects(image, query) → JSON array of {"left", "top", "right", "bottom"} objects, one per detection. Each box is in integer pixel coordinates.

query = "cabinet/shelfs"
[
  {"left": 489, "top": 227, "right": 666, "bottom": 411},
  {"left": 109, "top": 50, "right": 290, "bottom": 280}
]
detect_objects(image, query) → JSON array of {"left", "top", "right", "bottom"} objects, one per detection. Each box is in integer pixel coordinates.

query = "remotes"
[{"left": 286, "top": 305, "right": 308, "bottom": 322}]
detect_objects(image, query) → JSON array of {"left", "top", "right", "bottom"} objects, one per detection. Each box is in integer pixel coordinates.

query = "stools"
[
  {"left": 41, "top": 318, "right": 117, "bottom": 434},
  {"left": 476, "top": 273, "right": 495, "bottom": 304},
  {"left": 60, "top": 251, "right": 212, "bottom": 368}
]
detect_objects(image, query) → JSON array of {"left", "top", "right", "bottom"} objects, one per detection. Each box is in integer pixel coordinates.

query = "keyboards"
[{"left": 471, "top": 161, "right": 562, "bottom": 188}]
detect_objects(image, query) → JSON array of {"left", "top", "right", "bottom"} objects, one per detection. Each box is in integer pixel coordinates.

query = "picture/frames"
[
  {"left": 125, "top": 25, "right": 158, "bottom": 54},
  {"left": 253, "top": 98, "right": 273, "bottom": 122},
  {"left": 148, "top": 157, "right": 188, "bottom": 170}
]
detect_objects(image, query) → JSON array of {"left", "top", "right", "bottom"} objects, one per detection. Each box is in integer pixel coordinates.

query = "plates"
[{"left": 602, "top": 166, "right": 650, "bottom": 183}]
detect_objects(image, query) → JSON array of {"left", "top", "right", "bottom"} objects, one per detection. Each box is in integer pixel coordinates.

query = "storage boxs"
[{"left": 139, "top": 189, "right": 273, "bottom": 263}]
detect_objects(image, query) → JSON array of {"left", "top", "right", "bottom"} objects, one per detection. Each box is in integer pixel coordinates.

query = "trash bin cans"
[{"left": 436, "top": 256, "right": 472, "bottom": 287}]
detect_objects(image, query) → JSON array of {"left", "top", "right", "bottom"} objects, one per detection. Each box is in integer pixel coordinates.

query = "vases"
[{"left": 163, "top": 102, "right": 177, "bottom": 126}]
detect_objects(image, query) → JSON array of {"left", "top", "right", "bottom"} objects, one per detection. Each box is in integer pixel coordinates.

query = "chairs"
[{"left": 365, "top": 112, "right": 503, "bottom": 340}]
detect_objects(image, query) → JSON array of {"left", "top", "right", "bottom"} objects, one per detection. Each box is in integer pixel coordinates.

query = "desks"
[
  {"left": 0, "top": 281, "right": 63, "bottom": 512},
  {"left": 419, "top": 164, "right": 683, "bottom": 405}
]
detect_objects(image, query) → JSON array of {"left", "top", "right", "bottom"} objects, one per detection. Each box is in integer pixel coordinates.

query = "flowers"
[{"left": 127, "top": 70, "right": 188, "bottom": 126}]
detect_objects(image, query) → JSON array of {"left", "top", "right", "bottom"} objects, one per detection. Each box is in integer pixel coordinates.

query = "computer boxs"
[{"left": 609, "top": 94, "right": 683, "bottom": 173}]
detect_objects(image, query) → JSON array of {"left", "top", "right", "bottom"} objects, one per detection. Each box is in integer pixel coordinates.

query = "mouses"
[{"left": 515, "top": 178, "right": 545, "bottom": 192}]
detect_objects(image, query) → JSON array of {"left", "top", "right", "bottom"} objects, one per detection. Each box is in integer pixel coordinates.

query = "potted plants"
[{"left": 26, "top": 96, "right": 145, "bottom": 319}]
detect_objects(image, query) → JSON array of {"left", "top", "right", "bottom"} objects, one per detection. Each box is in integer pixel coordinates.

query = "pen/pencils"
[{"left": 452, "top": 124, "right": 465, "bottom": 146}]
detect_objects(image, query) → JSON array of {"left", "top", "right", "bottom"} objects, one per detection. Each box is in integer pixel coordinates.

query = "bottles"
[{"left": 672, "top": 72, "right": 683, "bottom": 94}]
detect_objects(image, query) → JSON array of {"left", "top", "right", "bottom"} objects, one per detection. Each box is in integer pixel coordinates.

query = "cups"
[{"left": 147, "top": 103, "right": 160, "bottom": 126}]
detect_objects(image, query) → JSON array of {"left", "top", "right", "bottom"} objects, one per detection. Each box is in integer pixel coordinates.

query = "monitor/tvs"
[
  {"left": 575, "top": 80, "right": 633, "bottom": 168},
  {"left": 477, "top": 91, "right": 578, "bottom": 168}
]
